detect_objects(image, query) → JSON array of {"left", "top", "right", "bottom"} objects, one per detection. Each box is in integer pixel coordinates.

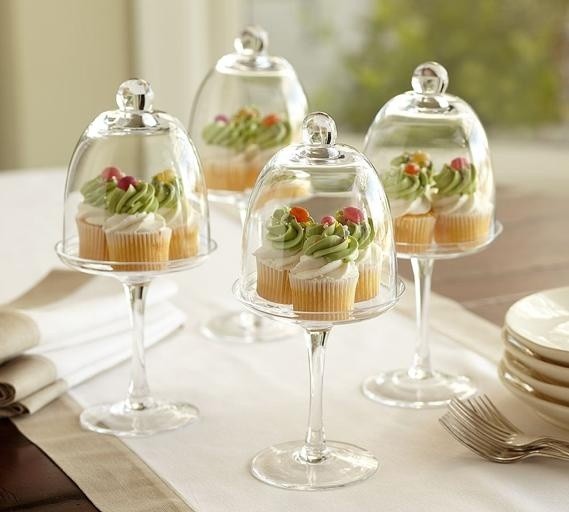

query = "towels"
[{"left": 1, "top": 296, "right": 188, "bottom": 421}]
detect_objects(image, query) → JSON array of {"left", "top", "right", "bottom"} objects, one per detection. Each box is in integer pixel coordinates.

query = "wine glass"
[
  {"left": 364, "top": 214, "right": 505, "bottom": 409},
  {"left": 188, "top": 186, "right": 303, "bottom": 343},
  {"left": 55, "top": 241, "right": 219, "bottom": 436},
  {"left": 233, "top": 272, "right": 404, "bottom": 493}
]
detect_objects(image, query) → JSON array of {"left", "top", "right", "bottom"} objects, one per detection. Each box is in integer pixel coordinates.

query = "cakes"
[
  {"left": 200, "top": 105, "right": 301, "bottom": 191},
  {"left": 77, "top": 166, "right": 200, "bottom": 273},
  {"left": 383, "top": 149, "right": 492, "bottom": 254},
  {"left": 254, "top": 205, "right": 384, "bottom": 321}
]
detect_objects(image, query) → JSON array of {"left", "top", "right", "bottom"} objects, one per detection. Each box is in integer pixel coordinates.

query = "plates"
[{"left": 498, "top": 284, "right": 569, "bottom": 433}]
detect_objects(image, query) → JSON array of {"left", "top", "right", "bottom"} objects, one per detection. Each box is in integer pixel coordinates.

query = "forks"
[{"left": 441, "top": 391, "right": 569, "bottom": 465}]
